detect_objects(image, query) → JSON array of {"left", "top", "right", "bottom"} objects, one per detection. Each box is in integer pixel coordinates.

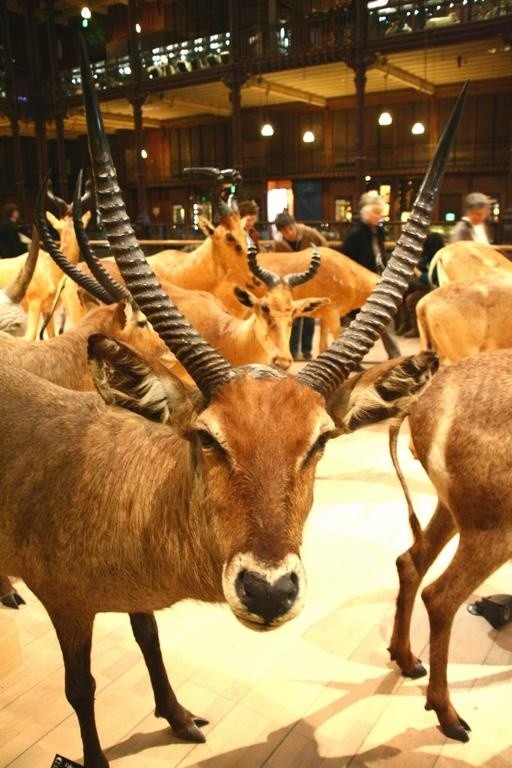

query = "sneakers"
[{"left": 290, "top": 350, "right": 314, "bottom": 359}]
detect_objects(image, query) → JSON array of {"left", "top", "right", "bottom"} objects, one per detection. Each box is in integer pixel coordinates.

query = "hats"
[
  {"left": 464, "top": 193, "right": 497, "bottom": 207},
  {"left": 276, "top": 214, "right": 294, "bottom": 230}
]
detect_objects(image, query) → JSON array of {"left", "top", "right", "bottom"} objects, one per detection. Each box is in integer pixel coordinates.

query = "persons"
[
  {"left": 0, "top": 202, "right": 34, "bottom": 258},
  {"left": 272, "top": 212, "right": 330, "bottom": 365},
  {"left": 411, "top": 265, "right": 436, "bottom": 284},
  {"left": 448, "top": 192, "right": 512, "bottom": 261},
  {"left": 235, "top": 197, "right": 262, "bottom": 256},
  {"left": 394, "top": 230, "right": 443, "bottom": 338},
  {"left": 339, "top": 189, "right": 390, "bottom": 320}
]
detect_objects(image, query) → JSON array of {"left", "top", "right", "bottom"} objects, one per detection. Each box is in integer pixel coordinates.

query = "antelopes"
[{"left": 0, "top": 20, "right": 512, "bottom": 768}]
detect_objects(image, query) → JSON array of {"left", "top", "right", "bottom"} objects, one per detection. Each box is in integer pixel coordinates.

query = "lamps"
[
  {"left": 304, "top": 105, "right": 315, "bottom": 142},
  {"left": 378, "top": 74, "right": 392, "bottom": 126},
  {"left": 261, "top": 86, "right": 275, "bottom": 137},
  {"left": 411, "top": 89, "right": 425, "bottom": 134}
]
